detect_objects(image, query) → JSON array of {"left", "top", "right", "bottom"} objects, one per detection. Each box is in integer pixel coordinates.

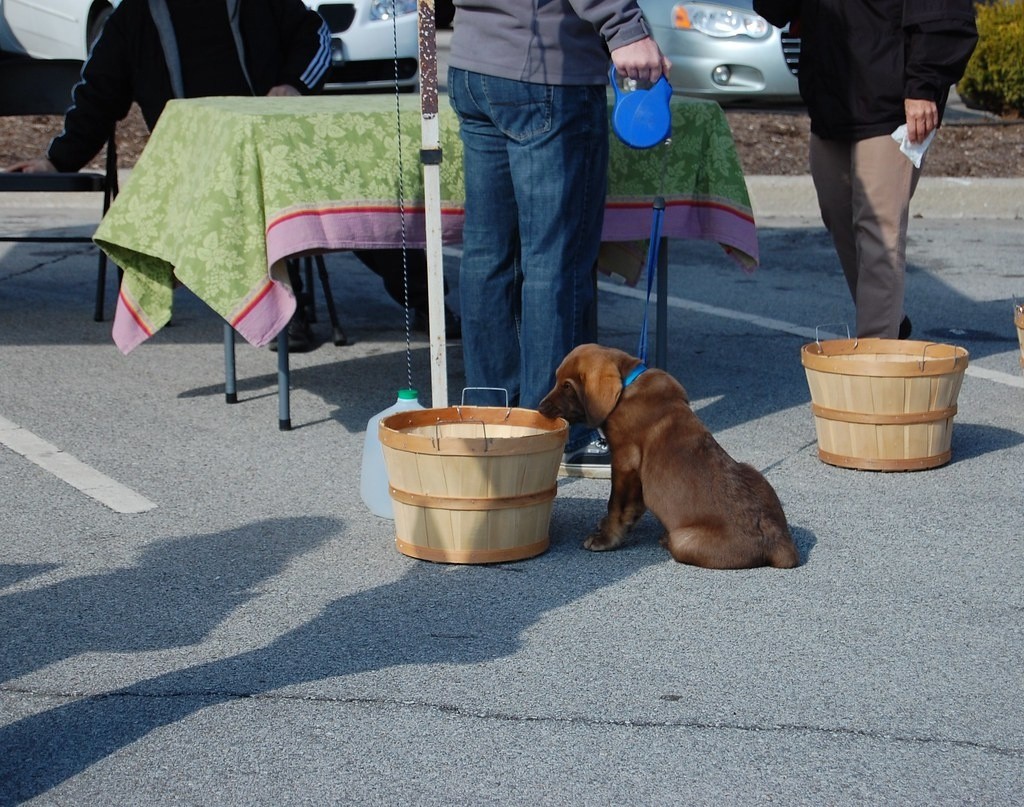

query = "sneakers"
[
  {"left": 268, "top": 271, "right": 314, "bottom": 353},
  {"left": 411, "top": 300, "right": 462, "bottom": 339},
  {"left": 559, "top": 436, "right": 613, "bottom": 479}
]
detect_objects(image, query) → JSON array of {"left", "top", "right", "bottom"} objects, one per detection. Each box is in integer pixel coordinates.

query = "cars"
[
  {"left": 635, "top": 1, "right": 804, "bottom": 97},
  {"left": 1, "top": 0, "right": 419, "bottom": 97}
]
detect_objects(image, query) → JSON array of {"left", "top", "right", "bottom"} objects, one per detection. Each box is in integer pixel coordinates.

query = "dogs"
[{"left": 536, "top": 343, "right": 800, "bottom": 571}]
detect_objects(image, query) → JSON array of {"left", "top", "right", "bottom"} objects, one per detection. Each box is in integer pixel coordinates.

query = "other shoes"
[{"left": 898, "top": 315, "right": 912, "bottom": 340}]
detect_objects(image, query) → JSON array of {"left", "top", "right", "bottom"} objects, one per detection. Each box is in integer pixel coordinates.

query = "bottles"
[{"left": 360, "top": 389, "right": 426, "bottom": 519}]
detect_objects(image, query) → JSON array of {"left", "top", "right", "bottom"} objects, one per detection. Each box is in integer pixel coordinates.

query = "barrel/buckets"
[
  {"left": 800, "top": 323, "right": 969, "bottom": 471},
  {"left": 379, "top": 387, "right": 569, "bottom": 563}
]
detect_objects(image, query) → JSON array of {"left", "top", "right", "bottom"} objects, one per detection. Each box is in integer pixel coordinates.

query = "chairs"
[{"left": 0, "top": 0, "right": 124, "bottom": 323}]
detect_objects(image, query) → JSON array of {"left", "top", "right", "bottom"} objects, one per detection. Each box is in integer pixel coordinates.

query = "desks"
[{"left": 91, "top": 84, "right": 760, "bottom": 434}]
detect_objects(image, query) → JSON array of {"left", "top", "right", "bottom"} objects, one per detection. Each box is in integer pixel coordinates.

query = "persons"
[
  {"left": 751, "top": 0, "right": 980, "bottom": 340},
  {"left": 449, "top": 0, "right": 673, "bottom": 479},
  {"left": 3, "top": 0, "right": 461, "bottom": 300}
]
